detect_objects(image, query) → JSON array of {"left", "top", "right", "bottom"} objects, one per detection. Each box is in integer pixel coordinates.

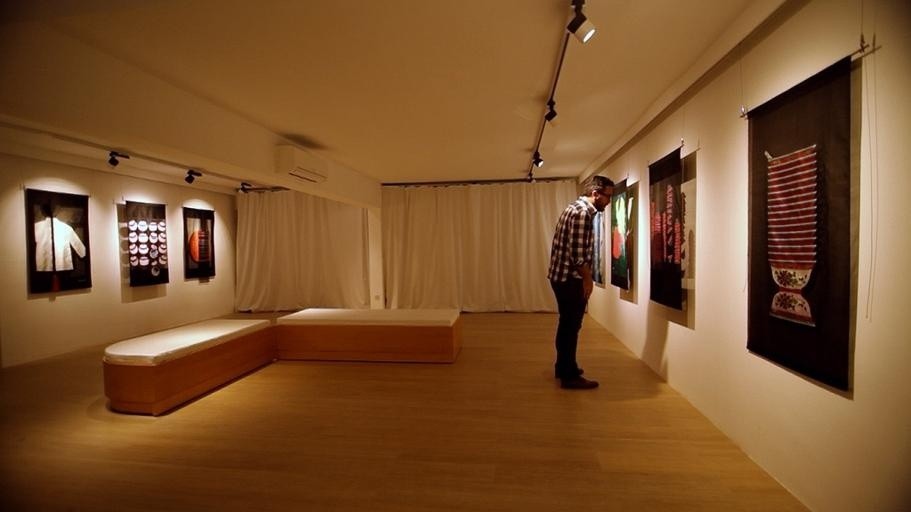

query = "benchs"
[
  {"left": 276, "top": 307, "right": 462, "bottom": 366},
  {"left": 100, "top": 317, "right": 271, "bottom": 417}
]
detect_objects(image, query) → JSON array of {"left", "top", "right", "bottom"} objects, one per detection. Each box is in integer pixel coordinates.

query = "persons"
[{"left": 547, "top": 176, "right": 613, "bottom": 389}]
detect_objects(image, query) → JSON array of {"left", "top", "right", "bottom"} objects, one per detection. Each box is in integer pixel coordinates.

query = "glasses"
[{"left": 596, "top": 190, "right": 612, "bottom": 199}]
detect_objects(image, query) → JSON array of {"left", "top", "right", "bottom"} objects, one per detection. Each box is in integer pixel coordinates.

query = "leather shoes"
[{"left": 555, "top": 367, "right": 599, "bottom": 389}]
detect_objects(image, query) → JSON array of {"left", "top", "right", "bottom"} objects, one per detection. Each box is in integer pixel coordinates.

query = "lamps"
[
  {"left": 562, "top": 0, "right": 596, "bottom": 42},
  {"left": 185, "top": 169, "right": 203, "bottom": 185},
  {"left": 107, "top": 151, "right": 130, "bottom": 169}
]
